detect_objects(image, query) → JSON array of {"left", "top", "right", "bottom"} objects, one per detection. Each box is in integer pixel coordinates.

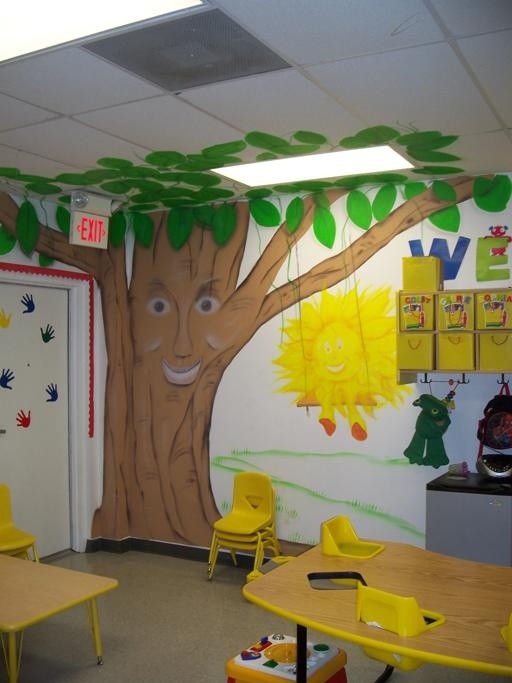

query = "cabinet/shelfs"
[{"left": 394, "top": 287, "right": 512, "bottom": 384}]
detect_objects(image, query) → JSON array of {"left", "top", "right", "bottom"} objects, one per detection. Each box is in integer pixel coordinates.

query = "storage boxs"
[{"left": 402, "top": 256, "right": 443, "bottom": 291}]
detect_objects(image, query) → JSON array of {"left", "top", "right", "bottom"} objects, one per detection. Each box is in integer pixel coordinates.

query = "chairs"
[
  {"left": 0, "top": 483, "right": 40, "bottom": 562},
  {"left": 208, "top": 472, "right": 283, "bottom": 582}
]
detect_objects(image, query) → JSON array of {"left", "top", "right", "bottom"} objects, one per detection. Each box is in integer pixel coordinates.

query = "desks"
[
  {"left": 242, "top": 539, "right": 512, "bottom": 683},
  {"left": 425, "top": 469, "right": 512, "bottom": 568},
  {"left": 0, "top": 552, "right": 119, "bottom": 683}
]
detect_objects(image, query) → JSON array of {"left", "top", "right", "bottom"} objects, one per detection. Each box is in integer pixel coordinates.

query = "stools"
[{"left": 225, "top": 632, "right": 349, "bottom": 683}]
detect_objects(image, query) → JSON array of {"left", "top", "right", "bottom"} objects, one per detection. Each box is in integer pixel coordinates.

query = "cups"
[{"left": 448, "top": 462, "right": 468, "bottom": 477}]
